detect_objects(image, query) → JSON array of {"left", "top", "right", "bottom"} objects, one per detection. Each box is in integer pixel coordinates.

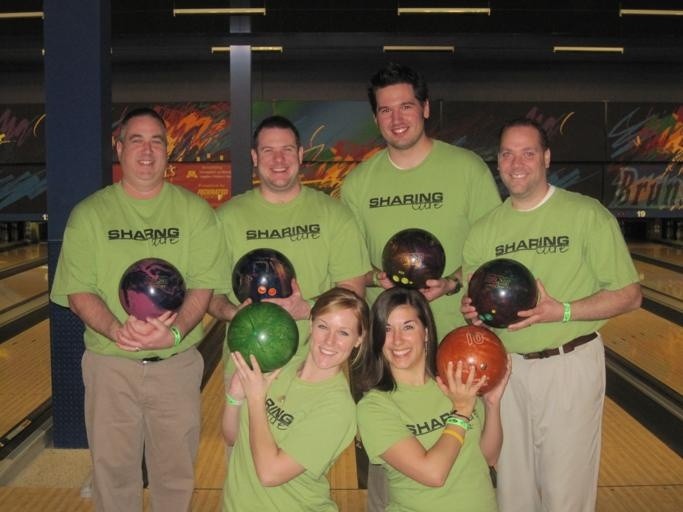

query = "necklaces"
[{"left": 279, "top": 364, "right": 303, "bottom": 404}]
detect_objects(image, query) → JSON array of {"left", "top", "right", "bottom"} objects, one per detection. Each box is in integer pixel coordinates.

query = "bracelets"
[
  {"left": 451, "top": 409, "right": 472, "bottom": 421},
  {"left": 443, "top": 415, "right": 473, "bottom": 430},
  {"left": 443, "top": 275, "right": 462, "bottom": 297},
  {"left": 441, "top": 427, "right": 464, "bottom": 445},
  {"left": 563, "top": 299, "right": 574, "bottom": 324},
  {"left": 225, "top": 391, "right": 240, "bottom": 408}
]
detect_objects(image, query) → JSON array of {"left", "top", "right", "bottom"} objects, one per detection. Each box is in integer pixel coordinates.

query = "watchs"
[{"left": 167, "top": 321, "right": 183, "bottom": 347}]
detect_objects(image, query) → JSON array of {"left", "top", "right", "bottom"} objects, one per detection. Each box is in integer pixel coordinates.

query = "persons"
[
  {"left": 48, "top": 103, "right": 221, "bottom": 508},
  {"left": 458, "top": 118, "right": 642, "bottom": 512},
  {"left": 216, "top": 286, "right": 372, "bottom": 511},
  {"left": 336, "top": 54, "right": 503, "bottom": 378},
  {"left": 201, "top": 107, "right": 372, "bottom": 380},
  {"left": 355, "top": 284, "right": 512, "bottom": 512}
]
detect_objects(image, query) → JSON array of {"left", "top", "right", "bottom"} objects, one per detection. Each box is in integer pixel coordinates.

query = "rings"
[
  {"left": 528, "top": 324, "right": 531, "bottom": 327},
  {"left": 135, "top": 347, "right": 140, "bottom": 351}
]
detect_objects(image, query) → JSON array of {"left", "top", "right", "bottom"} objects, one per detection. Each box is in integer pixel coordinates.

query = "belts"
[
  {"left": 139, "top": 352, "right": 179, "bottom": 363},
  {"left": 517, "top": 331, "right": 599, "bottom": 360}
]
